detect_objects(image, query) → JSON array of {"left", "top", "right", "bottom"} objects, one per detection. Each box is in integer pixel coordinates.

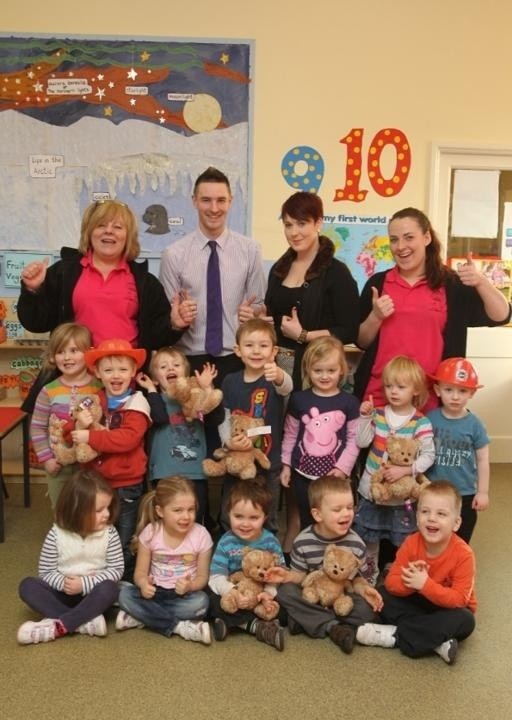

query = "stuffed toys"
[
  {"left": 220, "top": 546, "right": 281, "bottom": 621},
  {"left": 300, "top": 543, "right": 360, "bottom": 616}
]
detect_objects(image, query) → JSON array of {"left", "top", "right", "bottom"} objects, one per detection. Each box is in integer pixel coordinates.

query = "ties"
[{"left": 205, "top": 240, "right": 225, "bottom": 358}]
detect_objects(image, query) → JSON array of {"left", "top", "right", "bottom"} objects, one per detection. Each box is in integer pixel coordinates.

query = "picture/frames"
[{"left": 0, "top": 32, "right": 256, "bottom": 298}]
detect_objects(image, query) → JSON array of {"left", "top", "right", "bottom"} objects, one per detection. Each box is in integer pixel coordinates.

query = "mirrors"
[{"left": 446, "top": 164, "right": 512, "bottom": 261}]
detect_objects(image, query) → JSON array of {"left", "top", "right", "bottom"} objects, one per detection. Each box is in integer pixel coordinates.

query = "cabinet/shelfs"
[
  {"left": 0, "top": 298, "right": 49, "bottom": 484},
  {"left": 429, "top": 141, "right": 512, "bottom": 463}
]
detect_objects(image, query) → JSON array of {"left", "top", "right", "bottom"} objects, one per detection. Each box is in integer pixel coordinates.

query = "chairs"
[{"left": 0, "top": 407, "right": 29, "bottom": 543}]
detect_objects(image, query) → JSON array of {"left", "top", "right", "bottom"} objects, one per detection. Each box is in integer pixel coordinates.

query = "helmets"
[
  {"left": 426, "top": 356, "right": 484, "bottom": 390},
  {"left": 84, "top": 339, "right": 148, "bottom": 371}
]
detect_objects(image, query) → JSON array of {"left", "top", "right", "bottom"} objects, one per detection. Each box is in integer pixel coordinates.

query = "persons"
[
  {"left": 352, "top": 208, "right": 512, "bottom": 472},
  {"left": 114, "top": 476, "right": 214, "bottom": 646},
  {"left": 16, "top": 199, "right": 199, "bottom": 414},
  {"left": 256, "top": 191, "right": 360, "bottom": 397},
  {"left": 160, "top": 168, "right": 268, "bottom": 457},
  {"left": 208, "top": 479, "right": 286, "bottom": 652},
  {"left": 355, "top": 481, "right": 476, "bottom": 665},
  {"left": 265, "top": 476, "right": 385, "bottom": 654},
  {"left": 29, "top": 320, "right": 491, "bottom": 572},
  {"left": 15, "top": 467, "right": 126, "bottom": 644}
]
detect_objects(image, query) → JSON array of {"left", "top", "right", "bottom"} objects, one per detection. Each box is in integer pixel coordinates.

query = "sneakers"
[
  {"left": 328, "top": 621, "right": 355, "bottom": 654},
  {"left": 16, "top": 616, "right": 62, "bottom": 647},
  {"left": 77, "top": 612, "right": 108, "bottom": 639},
  {"left": 355, "top": 622, "right": 398, "bottom": 649},
  {"left": 432, "top": 637, "right": 458, "bottom": 664},
  {"left": 177, "top": 619, "right": 213, "bottom": 646},
  {"left": 114, "top": 609, "right": 145, "bottom": 631},
  {"left": 214, "top": 618, "right": 237, "bottom": 642},
  {"left": 288, "top": 615, "right": 306, "bottom": 635},
  {"left": 254, "top": 618, "right": 286, "bottom": 652}
]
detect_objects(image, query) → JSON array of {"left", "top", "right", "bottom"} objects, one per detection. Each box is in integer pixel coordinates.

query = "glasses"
[{"left": 86, "top": 199, "right": 129, "bottom": 232}]
